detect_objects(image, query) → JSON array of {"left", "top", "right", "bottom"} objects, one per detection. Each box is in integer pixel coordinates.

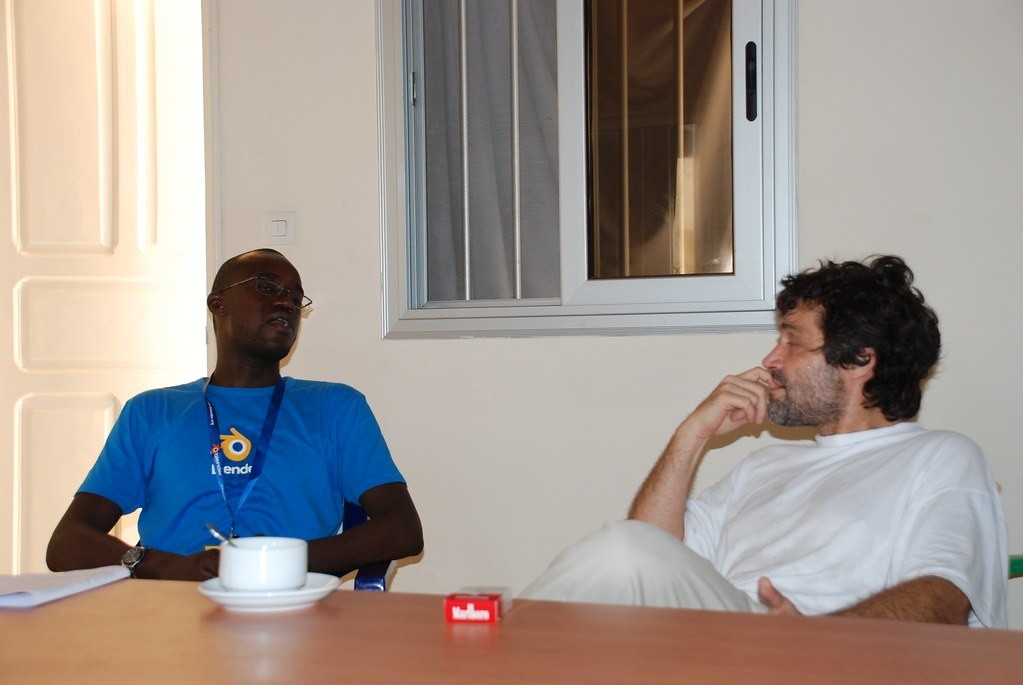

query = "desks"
[{"left": 0, "top": 579, "right": 1023, "bottom": 685}]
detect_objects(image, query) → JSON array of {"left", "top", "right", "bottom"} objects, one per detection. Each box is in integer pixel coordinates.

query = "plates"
[{"left": 199, "top": 572, "right": 342, "bottom": 611}]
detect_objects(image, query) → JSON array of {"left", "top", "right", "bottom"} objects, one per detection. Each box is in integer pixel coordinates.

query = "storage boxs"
[{"left": 444, "top": 586, "right": 513, "bottom": 626}]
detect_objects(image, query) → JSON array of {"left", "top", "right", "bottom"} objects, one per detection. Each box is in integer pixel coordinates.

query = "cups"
[{"left": 219, "top": 537, "right": 307, "bottom": 592}]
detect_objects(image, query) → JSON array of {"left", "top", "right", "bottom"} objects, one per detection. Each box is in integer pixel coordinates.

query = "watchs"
[{"left": 121, "top": 546, "right": 152, "bottom": 579}]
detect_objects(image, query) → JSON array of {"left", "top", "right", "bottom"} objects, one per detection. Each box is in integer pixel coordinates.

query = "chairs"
[{"left": 341, "top": 548, "right": 426, "bottom": 592}]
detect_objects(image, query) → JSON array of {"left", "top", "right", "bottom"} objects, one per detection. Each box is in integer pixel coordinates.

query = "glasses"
[{"left": 219, "top": 276, "right": 313, "bottom": 310}]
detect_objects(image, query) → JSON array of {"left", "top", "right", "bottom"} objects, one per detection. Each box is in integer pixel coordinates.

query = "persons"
[
  {"left": 523, "top": 255, "right": 1009, "bottom": 629},
  {"left": 45, "top": 248, "right": 425, "bottom": 582}
]
